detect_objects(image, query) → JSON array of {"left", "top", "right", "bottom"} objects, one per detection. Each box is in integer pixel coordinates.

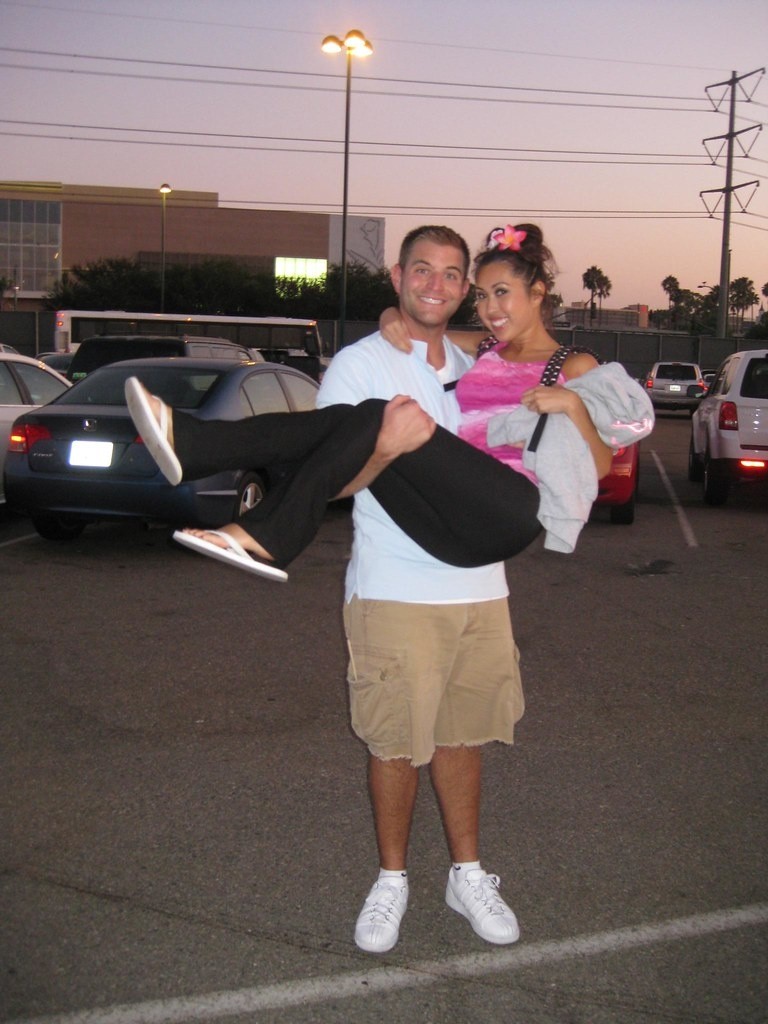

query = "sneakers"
[
  {"left": 445, "top": 865, "right": 522, "bottom": 945},
  {"left": 353, "top": 878, "right": 410, "bottom": 954}
]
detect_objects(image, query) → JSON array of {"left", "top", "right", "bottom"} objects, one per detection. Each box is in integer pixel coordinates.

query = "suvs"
[{"left": 68, "top": 334, "right": 265, "bottom": 385}]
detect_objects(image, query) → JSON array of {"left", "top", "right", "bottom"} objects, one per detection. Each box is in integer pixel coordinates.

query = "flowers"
[{"left": 493, "top": 223, "right": 528, "bottom": 251}]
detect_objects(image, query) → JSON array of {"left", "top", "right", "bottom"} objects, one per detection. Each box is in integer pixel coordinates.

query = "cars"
[
  {"left": 0, "top": 343, "right": 20, "bottom": 355},
  {"left": 686, "top": 349, "right": 768, "bottom": 504},
  {"left": 704, "top": 374, "right": 716, "bottom": 388},
  {"left": 35, "top": 352, "right": 74, "bottom": 379},
  {"left": 639, "top": 362, "right": 709, "bottom": 414},
  {"left": 3, "top": 358, "right": 352, "bottom": 540},
  {"left": 0, "top": 353, "right": 74, "bottom": 506},
  {"left": 701, "top": 368, "right": 718, "bottom": 377},
  {"left": 594, "top": 440, "right": 640, "bottom": 525}
]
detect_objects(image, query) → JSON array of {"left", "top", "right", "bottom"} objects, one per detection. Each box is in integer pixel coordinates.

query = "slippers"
[
  {"left": 172, "top": 528, "right": 290, "bottom": 583},
  {"left": 123, "top": 375, "right": 183, "bottom": 488}
]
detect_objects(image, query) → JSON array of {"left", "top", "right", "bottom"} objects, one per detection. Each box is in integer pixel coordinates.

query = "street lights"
[
  {"left": 320, "top": 30, "right": 374, "bottom": 350},
  {"left": 159, "top": 184, "right": 172, "bottom": 313}
]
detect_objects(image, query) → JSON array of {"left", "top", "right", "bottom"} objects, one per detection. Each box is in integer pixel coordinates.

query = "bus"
[{"left": 54, "top": 310, "right": 324, "bottom": 360}]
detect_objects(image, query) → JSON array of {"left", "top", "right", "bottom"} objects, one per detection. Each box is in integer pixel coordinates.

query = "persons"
[
  {"left": 124, "top": 222, "right": 613, "bottom": 583},
  {"left": 316, "top": 226, "right": 525, "bottom": 953}
]
